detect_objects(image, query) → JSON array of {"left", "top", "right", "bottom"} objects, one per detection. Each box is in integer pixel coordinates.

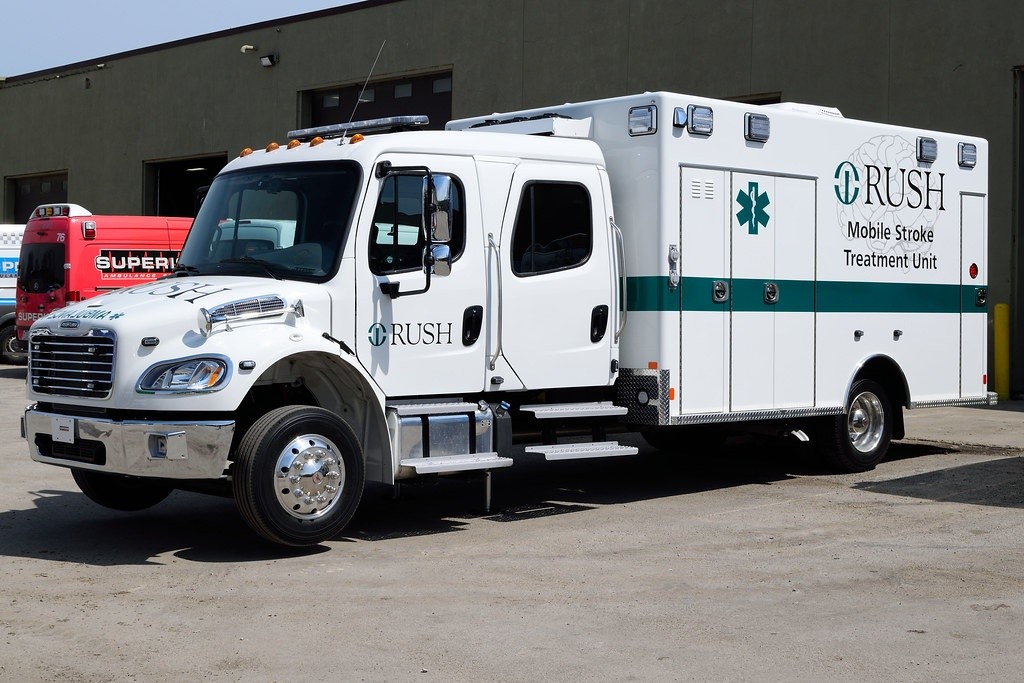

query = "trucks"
[{"left": 19, "top": 90, "right": 998, "bottom": 551}]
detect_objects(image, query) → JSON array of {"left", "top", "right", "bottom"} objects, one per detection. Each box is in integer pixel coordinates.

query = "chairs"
[{"left": 317, "top": 207, "right": 379, "bottom": 260}]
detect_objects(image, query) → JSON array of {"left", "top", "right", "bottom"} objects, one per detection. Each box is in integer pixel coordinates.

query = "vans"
[
  {"left": 209, "top": 219, "right": 420, "bottom": 263},
  {"left": 0, "top": 224, "right": 29, "bottom": 365},
  {"left": 15, "top": 203, "right": 230, "bottom": 351}
]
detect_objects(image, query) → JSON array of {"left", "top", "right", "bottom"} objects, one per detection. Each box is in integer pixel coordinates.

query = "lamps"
[{"left": 260, "top": 52, "right": 279, "bottom": 67}]
